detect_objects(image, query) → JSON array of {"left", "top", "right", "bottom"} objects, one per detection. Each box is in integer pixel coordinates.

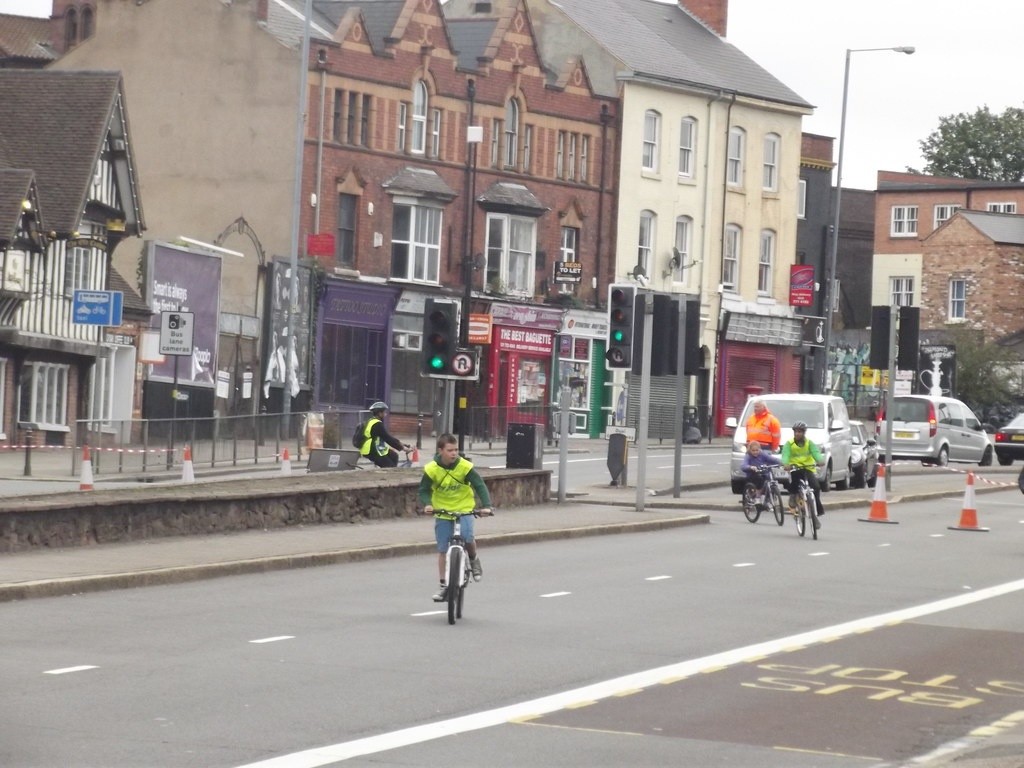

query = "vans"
[
  {"left": 873, "top": 395, "right": 995, "bottom": 468},
  {"left": 723, "top": 392, "right": 853, "bottom": 494}
]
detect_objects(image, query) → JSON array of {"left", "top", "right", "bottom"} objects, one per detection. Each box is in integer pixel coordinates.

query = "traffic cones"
[
  {"left": 947, "top": 472, "right": 990, "bottom": 532},
  {"left": 411, "top": 447, "right": 421, "bottom": 470},
  {"left": 80, "top": 446, "right": 96, "bottom": 492},
  {"left": 859, "top": 462, "right": 901, "bottom": 524},
  {"left": 181, "top": 446, "right": 195, "bottom": 482},
  {"left": 281, "top": 449, "right": 295, "bottom": 477}
]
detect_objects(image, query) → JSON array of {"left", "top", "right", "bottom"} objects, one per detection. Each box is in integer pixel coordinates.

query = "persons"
[
  {"left": 741, "top": 442, "right": 788, "bottom": 511},
  {"left": 419, "top": 433, "right": 491, "bottom": 600},
  {"left": 782, "top": 421, "right": 826, "bottom": 528},
  {"left": 746, "top": 401, "right": 783, "bottom": 512},
  {"left": 360, "top": 402, "right": 416, "bottom": 468}
]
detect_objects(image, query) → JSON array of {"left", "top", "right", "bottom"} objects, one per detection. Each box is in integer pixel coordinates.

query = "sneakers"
[
  {"left": 432, "top": 584, "right": 448, "bottom": 600},
  {"left": 468, "top": 556, "right": 483, "bottom": 582}
]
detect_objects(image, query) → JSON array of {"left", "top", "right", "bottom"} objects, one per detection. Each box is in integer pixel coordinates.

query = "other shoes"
[
  {"left": 766, "top": 502, "right": 774, "bottom": 512},
  {"left": 813, "top": 515, "right": 821, "bottom": 529},
  {"left": 754, "top": 497, "right": 762, "bottom": 507},
  {"left": 789, "top": 493, "right": 798, "bottom": 508}
]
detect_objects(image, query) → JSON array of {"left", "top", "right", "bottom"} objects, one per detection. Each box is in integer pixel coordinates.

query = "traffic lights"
[
  {"left": 421, "top": 296, "right": 462, "bottom": 379},
  {"left": 606, "top": 284, "right": 636, "bottom": 372}
]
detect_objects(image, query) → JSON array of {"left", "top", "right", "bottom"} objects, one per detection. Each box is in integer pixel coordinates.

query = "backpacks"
[{"left": 352, "top": 418, "right": 381, "bottom": 449}]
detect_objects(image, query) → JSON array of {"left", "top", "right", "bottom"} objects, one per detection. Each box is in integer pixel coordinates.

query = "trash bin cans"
[{"left": 506, "top": 422, "right": 545, "bottom": 470}]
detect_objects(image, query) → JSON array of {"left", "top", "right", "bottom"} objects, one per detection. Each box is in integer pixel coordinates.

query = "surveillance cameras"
[
  {"left": 717, "top": 284, "right": 723, "bottom": 295},
  {"left": 637, "top": 274, "right": 648, "bottom": 288}
]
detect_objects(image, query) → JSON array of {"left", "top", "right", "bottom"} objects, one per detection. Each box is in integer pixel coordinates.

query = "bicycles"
[
  {"left": 347, "top": 444, "right": 418, "bottom": 469},
  {"left": 783, "top": 461, "right": 826, "bottom": 541},
  {"left": 741, "top": 464, "right": 788, "bottom": 527},
  {"left": 417, "top": 507, "right": 495, "bottom": 623}
]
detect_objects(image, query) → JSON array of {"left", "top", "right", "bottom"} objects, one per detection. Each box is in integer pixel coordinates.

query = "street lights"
[{"left": 819, "top": 46, "right": 918, "bottom": 391}]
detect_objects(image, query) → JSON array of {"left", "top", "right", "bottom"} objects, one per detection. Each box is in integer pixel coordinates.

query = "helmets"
[
  {"left": 792, "top": 421, "right": 807, "bottom": 431},
  {"left": 369, "top": 401, "right": 388, "bottom": 411}
]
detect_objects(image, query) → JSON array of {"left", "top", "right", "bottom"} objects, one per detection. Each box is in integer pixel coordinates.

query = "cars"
[
  {"left": 994, "top": 412, "right": 1024, "bottom": 466},
  {"left": 848, "top": 420, "right": 881, "bottom": 490}
]
